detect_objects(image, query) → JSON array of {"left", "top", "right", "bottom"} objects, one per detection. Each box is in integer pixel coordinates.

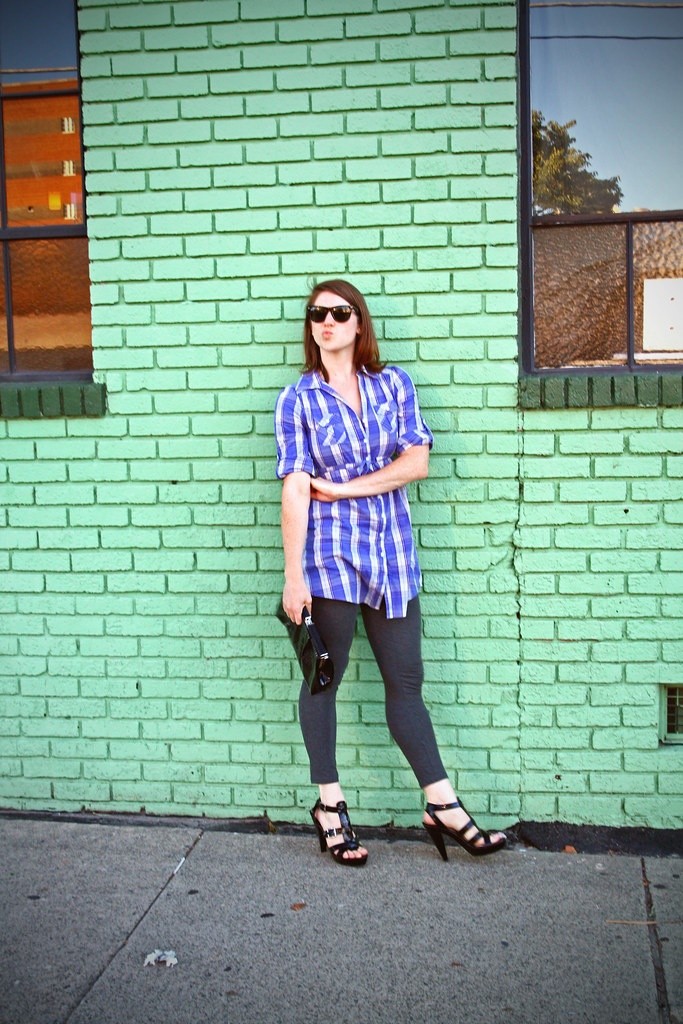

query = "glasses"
[{"left": 308, "top": 305, "right": 356, "bottom": 323}]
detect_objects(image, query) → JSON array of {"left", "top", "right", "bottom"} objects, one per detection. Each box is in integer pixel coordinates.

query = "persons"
[{"left": 273, "top": 280, "right": 508, "bottom": 868}]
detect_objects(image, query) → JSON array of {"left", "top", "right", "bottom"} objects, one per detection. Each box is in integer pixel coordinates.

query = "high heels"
[
  {"left": 309, "top": 798, "right": 367, "bottom": 866},
  {"left": 422, "top": 797, "right": 507, "bottom": 861}
]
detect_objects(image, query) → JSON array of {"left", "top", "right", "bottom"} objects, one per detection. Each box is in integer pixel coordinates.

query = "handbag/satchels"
[{"left": 275, "top": 598, "right": 334, "bottom": 694}]
